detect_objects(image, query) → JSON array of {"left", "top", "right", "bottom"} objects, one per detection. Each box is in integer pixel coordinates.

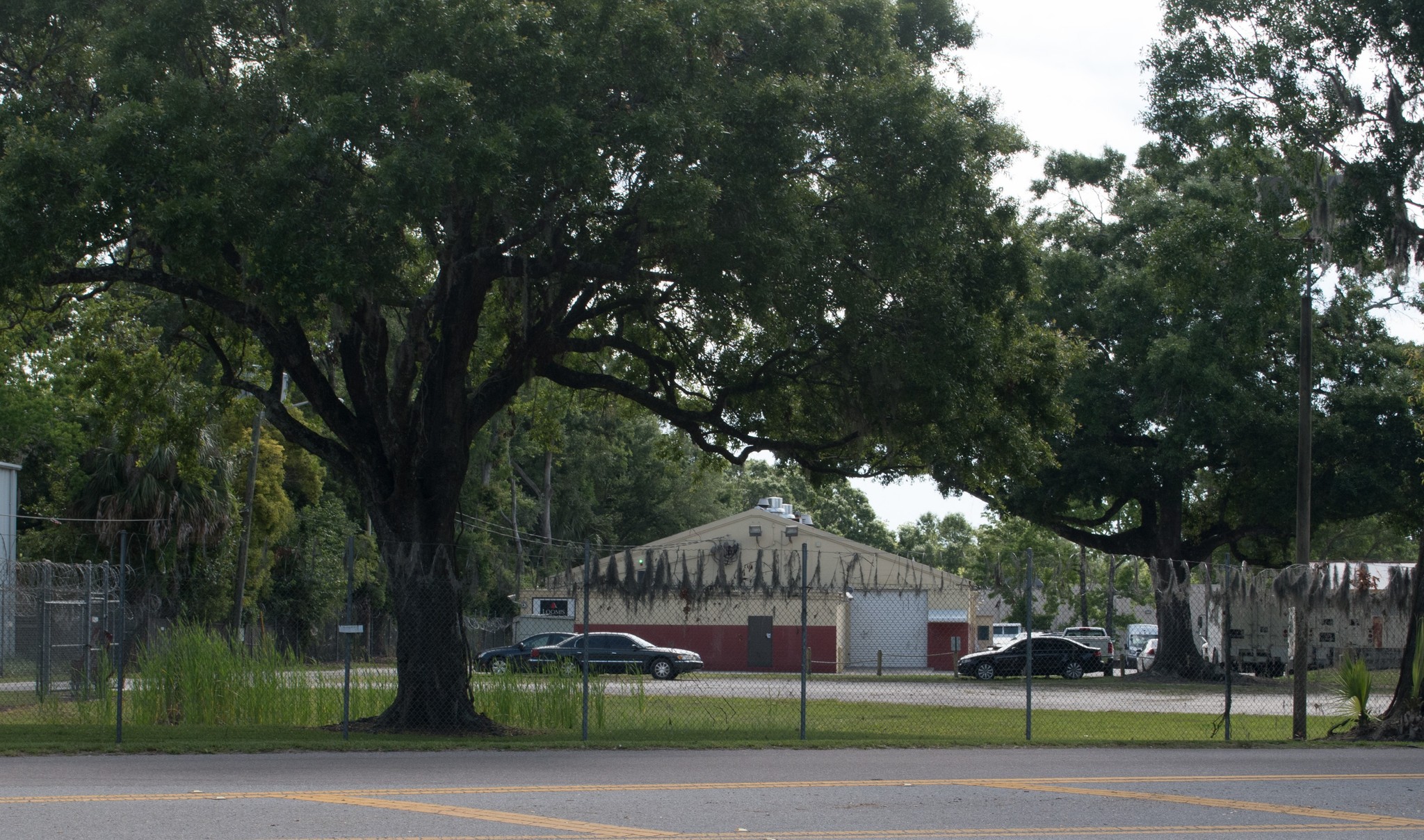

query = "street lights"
[
  {"left": 225, "top": 364, "right": 289, "bottom": 648},
  {"left": 1259, "top": 198, "right": 1370, "bottom": 743}
]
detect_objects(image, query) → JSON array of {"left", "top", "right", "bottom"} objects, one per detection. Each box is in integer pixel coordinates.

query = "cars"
[
  {"left": 473, "top": 632, "right": 579, "bottom": 677},
  {"left": 980, "top": 623, "right": 1063, "bottom": 652},
  {"left": 958, "top": 636, "right": 1105, "bottom": 681},
  {"left": 527, "top": 631, "right": 704, "bottom": 680},
  {"left": 1136, "top": 638, "right": 1159, "bottom": 672}
]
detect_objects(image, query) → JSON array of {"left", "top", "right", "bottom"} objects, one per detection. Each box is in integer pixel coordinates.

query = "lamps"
[
  {"left": 781, "top": 525, "right": 798, "bottom": 543},
  {"left": 749, "top": 525, "right": 763, "bottom": 543},
  {"left": 1145, "top": 610, "right": 1151, "bottom": 614}
]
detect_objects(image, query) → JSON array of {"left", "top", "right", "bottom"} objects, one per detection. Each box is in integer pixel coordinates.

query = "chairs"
[
  {"left": 1069, "top": 634, "right": 1076, "bottom": 636},
  {"left": 1094, "top": 634, "right": 1100, "bottom": 636},
  {"left": 552, "top": 637, "right": 564, "bottom": 645}
]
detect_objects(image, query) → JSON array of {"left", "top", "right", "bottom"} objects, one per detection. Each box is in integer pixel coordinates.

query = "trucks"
[
  {"left": 1197, "top": 591, "right": 1410, "bottom": 679},
  {"left": 1123, "top": 623, "right": 1158, "bottom": 669}
]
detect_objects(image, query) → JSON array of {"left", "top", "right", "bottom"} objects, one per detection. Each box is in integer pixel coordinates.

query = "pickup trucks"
[{"left": 1060, "top": 627, "right": 1116, "bottom": 676}]
